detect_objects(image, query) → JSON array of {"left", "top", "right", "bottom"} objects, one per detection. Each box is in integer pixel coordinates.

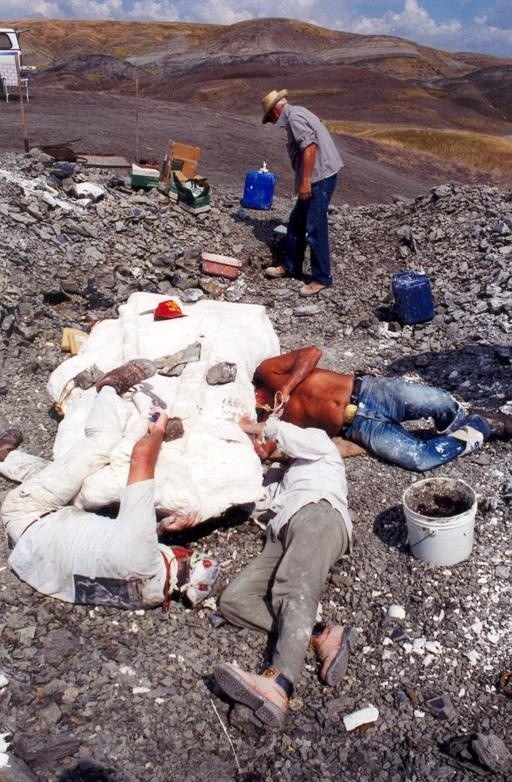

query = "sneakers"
[
  {"left": 95, "top": 358, "right": 158, "bottom": 397},
  {"left": 0, "top": 427, "right": 24, "bottom": 463}
]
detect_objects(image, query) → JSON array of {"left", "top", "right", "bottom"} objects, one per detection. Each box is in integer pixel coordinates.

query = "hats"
[
  {"left": 261, "top": 89, "right": 288, "bottom": 127},
  {"left": 154, "top": 299, "right": 186, "bottom": 321}
]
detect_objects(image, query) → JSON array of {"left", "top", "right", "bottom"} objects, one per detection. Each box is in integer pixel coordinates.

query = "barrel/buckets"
[
  {"left": 242, "top": 161, "right": 275, "bottom": 210},
  {"left": 392, "top": 272, "right": 434, "bottom": 323},
  {"left": 404, "top": 476, "right": 477, "bottom": 567}
]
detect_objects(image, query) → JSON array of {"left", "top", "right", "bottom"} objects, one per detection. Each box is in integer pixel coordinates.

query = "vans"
[{"left": 0, "top": 25, "right": 32, "bottom": 96}]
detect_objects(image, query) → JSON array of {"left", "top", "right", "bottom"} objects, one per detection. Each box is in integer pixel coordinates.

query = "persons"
[
  {"left": 0, "top": 358, "right": 222, "bottom": 610},
  {"left": 212, "top": 415, "right": 361, "bottom": 727},
  {"left": 252, "top": 346, "right": 511, "bottom": 473},
  {"left": 261, "top": 89, "right": 345, "bottom": 297}
]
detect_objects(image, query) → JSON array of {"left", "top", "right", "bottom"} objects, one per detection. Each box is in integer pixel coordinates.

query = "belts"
[
  {"left": 340, "top": 380, "right": 362, "bottom": 436},
  {"left": 20, "top": 508, "right": 56, "bottom": 531}
]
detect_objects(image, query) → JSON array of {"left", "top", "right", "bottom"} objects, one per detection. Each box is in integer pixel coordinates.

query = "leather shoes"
[
  {"left": 265, "top": 263, "right": 302, "bottom": 278},
  {"left": 213, "top": 657, "right": 295, "bottom": 728},
  {"left": 470, "top": 406, "right": 512, "bottom": 439},
  {"left": 299, "top": 280, "right": 334, "bottom": 297},
  {"left": 313, "top": 622, "right": 361, "bottom": 687}
]
